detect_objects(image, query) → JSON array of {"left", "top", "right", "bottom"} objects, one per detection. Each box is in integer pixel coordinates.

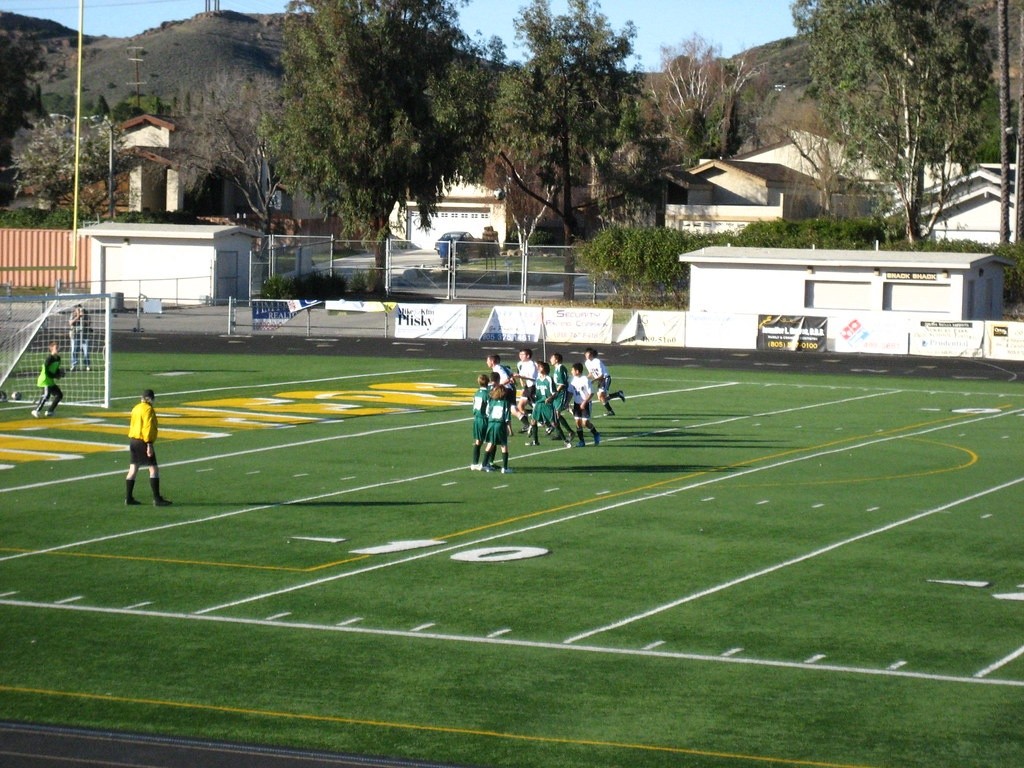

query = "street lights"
[
  {"left": 90, "top": 115, "right": 114, "bottom": 221},
  {"left": 1006, "top": 126, "right": 1019, "bottom": 243}
]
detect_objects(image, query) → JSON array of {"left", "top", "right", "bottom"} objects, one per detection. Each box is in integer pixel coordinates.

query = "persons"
[
  {"left": 31, "top": 342, "right": 65, "bottom": 420},
  {"left": 125, "top": 388, "right": 173, "bottom": 506},
  {"left": 470, "top": 348, "right": 625, "bottom": 473},
  {"left": 69, "top": 303, "right": 95, "bottom": 371}
]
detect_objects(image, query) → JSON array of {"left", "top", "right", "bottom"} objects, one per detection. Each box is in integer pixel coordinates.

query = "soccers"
[{"left": 11, "top": 391, "right": 21, "bottom": 400}]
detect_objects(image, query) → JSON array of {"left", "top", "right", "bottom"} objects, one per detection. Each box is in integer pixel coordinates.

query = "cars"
[{"left": 435, "top": 231, "right": 473, "bottom": 254}]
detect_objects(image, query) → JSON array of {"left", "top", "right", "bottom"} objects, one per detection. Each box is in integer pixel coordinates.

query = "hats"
[{"left": 143, "top": 389, "right": 154, "bottom": 397}]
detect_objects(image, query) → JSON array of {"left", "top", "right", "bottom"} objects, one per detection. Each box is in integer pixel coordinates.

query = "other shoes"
[
  {"left": 85, "top": 366, "right": 91, "bottom": 371},
  {"left": 544, "top": 426, "right": 554, "bottom": 437},
  {"left": 153, "top": 496, "right": 172, "bottom": 506},
  {"left": 518, "top": 426, "right": 529, "bottom": 432},
  {"left": 568, "top": 432, "right": 575, "bottom": 442},
  {"left": 551, "top": 435, "right": 561, "bottom": 440},
  {"left": 501, "top": 468, "right": 516, "bottom": 473},
  {"left": 594, "top": 433, "right": 601, "bottom": 446},
  {"left": 125, "top": 497, "right": 141, "bottom": 505},
  {"left": 471, "top": 463, "right": 482, "bottom": 471},
  {"left": 525, "top": 440, "right": 540, "bottom": 445},
  {"left": 604, "top": 411, "right": 615, "bottom": 417},
  {"left": 617, "top": 390, "right": 625, "bottom": 402},
  {"left": 31, "top": 410, "right": 42, "bottom": 419},
  {"left": 70, "top": 366, "right": 76, "bottom": 371},
  {"left": 564, "top": 440, "right": 572, "bottom": 449},
  {"left": 527, "top": 425, "right": 533, "bottom": 438},
  {"left": 576, "top": 441, "right": 585, "bottom": 447},
  {"left": 479, "top": 464, "right": 495, "bottom": 472},
  {"left": 45, "top": 411, "right": 55, "bottom": 417}
]
